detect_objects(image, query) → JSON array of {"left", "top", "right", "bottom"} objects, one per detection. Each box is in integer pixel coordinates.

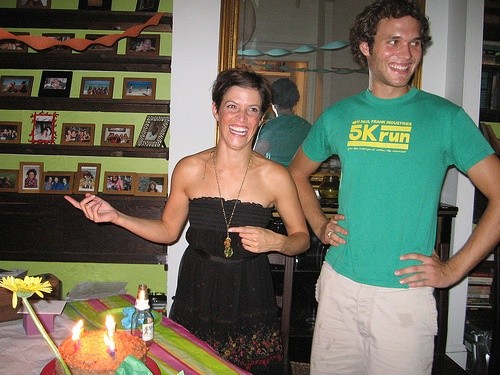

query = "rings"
[{"left": 327, "top": 231, "right": 333, "bottom": 238}]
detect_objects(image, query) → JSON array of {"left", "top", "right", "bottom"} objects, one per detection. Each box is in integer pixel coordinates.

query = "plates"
[
  {"left": 39, "top": 355, "right": 161, "bottom": 375},
  {"left": 96, "top": 307, "right": 162, "bottom": 331}
]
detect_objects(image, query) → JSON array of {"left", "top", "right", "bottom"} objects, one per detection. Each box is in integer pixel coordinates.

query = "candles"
[
  {"left": 103, "top": 313, "right": 118, "bottom": 339},
  {"left": 71, "top": 316, "right": 86, "bottom": 350},
  {"left": 100, "top": 333, "right": 118, "bottom": 359}
]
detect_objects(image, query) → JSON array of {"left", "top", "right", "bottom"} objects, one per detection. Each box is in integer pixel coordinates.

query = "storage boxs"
[
  {"left": 0, "top": 273, "right": 64, "bottom": 323},
  {"left": 24, "top": 312, "right": 56, "bottom": 336}
]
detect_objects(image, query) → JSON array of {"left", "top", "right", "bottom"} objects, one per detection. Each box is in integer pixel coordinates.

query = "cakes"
[{"left": 55, "top": 329, "right": 148, "bottom": 375}]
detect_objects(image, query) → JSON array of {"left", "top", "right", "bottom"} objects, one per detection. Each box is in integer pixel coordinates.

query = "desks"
[{"left": 0, "top": 292, "right": 252, "bottom": 375}]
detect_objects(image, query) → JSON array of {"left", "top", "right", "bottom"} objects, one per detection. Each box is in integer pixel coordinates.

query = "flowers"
[{"left": 0, "top": 275, "right": 71, "bottom": 375}]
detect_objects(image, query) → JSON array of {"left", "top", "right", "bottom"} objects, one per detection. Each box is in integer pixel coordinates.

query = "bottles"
[
  {"left": 318, "top": 175, "right": 341, "bottom": 198},
  {"left": 131, "top": 284, "right": 154, "bottom": 347}
]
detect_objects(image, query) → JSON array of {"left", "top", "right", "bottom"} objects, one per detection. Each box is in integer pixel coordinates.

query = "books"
[{"left": 466, "top": 271, "right": 495, "bottom": 309}]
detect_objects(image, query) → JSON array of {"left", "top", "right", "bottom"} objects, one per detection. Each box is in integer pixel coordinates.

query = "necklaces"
[{"left": 213, "top": 147, "right": 254, "bottom": 259}]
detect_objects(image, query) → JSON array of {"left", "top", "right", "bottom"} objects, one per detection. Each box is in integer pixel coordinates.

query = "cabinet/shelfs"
[
  {"left": 267, "top": 202, "right": 459, "bottom": 375},
  {"left": 0, "top": 5, "right": 176, "bottom": 272},
  {"left": 466, "top": 0, "right": 500, "bottom": 375}
]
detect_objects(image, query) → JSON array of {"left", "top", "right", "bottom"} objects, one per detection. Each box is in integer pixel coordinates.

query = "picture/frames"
[
  {"left": 0, "top": 120, "right": 22, "bottom": 144},
  {"left": 84, "top": 32, "right": 118, "bottom": 55},
  {"left": 42, "top": 33, "right": 76, "bottom": 53},
  {"left": 103, "top": 171, "right": 135, "bottom": 196},
  {"left": 15, "top": 0, "right": 160, "bottom": 12},
  {"left": 126, "top": 34, "right": 160, "bottom": 56},
  {"left": 122, "top": 77, "right": 157, "bottom": 99},
  {"left": 134, "top": 173, "right": 168, "bottom": 196},
  {"left": 28, "top": 111, "right": 60, "bottom": 144},
  {"left": 0, "top": 31, "right": 29, "bottom": 53},
  {"left": 38, "top": 70, "right": 73, "bottom": 97},
  {"left": 0, "top": 74, "right": 34, "bottom": 97},
  {"left": 134, "top": 115, "right": 170, "bottom": 148},
  {"left": 74, "top": 161, "right": 101, "bottom": 197},
  {"left": 0, "top": 168, "right": 20, "bottom": 192},
  {"left": 18, "top": 160, "right": 44, "bottom": 193},
  {"left": 39, "top": 171, "right": 75, "bottom": 194},
  {"left": 79, "top": 76, "right": 114, "bottom": 99},
  {"left": 59, "top": 122, "right": 96, "bottom": 145},
  {"left": 100, "top": 124, "right": 134, "bottom": 148}
]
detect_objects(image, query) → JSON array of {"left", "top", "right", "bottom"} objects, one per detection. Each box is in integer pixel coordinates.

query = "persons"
[
  {"left": 65, "top": 61, "right": 311, "bottom": 375},
  {"left": 253, "top": 77, "right": 314, "bottom": 168},
  {"left": 286, "top": 0, "right": 500, "bottom": 375},
  {"left": 0, "top": 79, "right": 160, "bottom": 192},
  {"left": 130, "top": 38, "right": 152, "bottom": 53}
]
